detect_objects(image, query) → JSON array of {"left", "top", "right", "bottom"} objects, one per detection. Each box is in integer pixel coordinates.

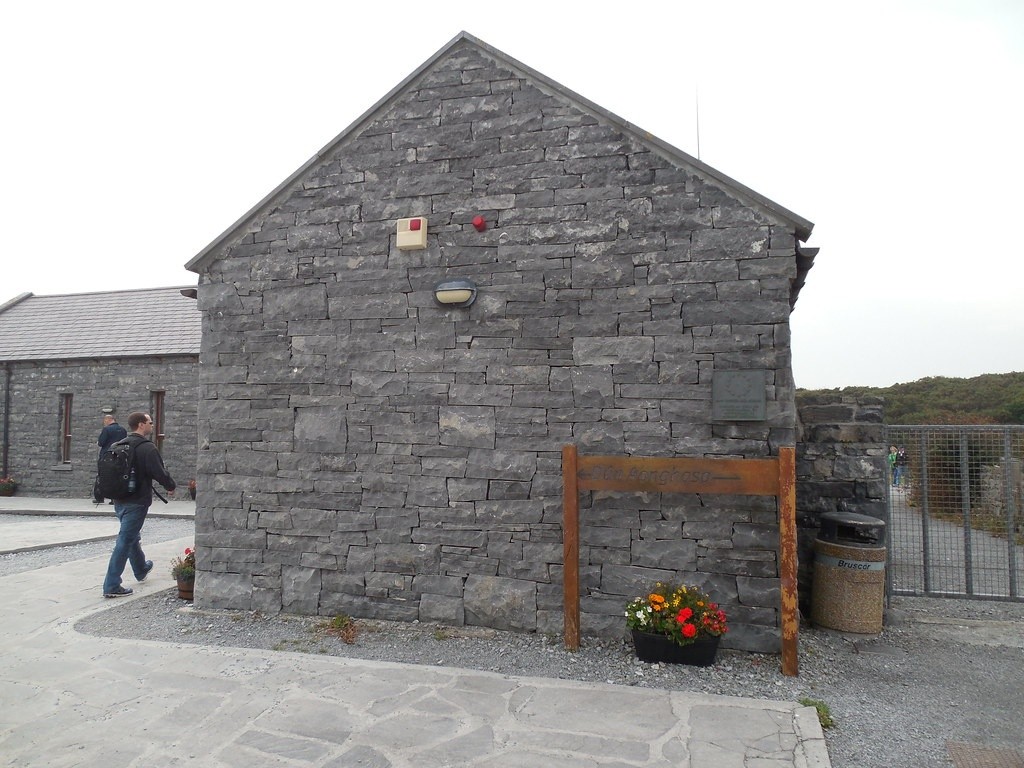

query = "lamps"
[{"left": 432, "top": 277, "right": 477, "bottom": 308}]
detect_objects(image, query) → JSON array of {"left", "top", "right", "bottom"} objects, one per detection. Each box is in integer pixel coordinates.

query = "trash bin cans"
[{"left": 810, "top": 506, "right": 889, "bottom": 637}]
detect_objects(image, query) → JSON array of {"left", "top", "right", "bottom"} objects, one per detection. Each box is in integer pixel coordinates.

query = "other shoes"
[{"left": 93, "top": 499, "right": 104, "bottom": 503}]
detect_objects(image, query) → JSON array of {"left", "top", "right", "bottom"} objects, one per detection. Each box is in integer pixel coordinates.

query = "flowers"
[
  {"left": 623, "top": 578, "right": 730, "bottom": 647},
  {"left": 188, "top": 480, "right": 196, "bottom": 493},
  {"left": 170, "top": 548, "right": 195, "bottom": 584},
  {"left": 0, "top": 473, "right": 21, "bottom": 492}
]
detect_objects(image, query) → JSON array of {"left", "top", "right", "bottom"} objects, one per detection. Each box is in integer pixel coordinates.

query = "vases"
[
  {"left": 176, "top": 575, "right": 193, "bottom": 600},
  {"left": 0, "top": 488, "right": 16, "bottom": 496},
  {"left": 630, "top": 629, "right": 721, "bottom": 667},
  {"left": 191, "top": 493, "right": 196, "bottom": 500}
]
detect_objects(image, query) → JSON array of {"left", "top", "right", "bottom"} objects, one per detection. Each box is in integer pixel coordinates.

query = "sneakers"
[
  {"left": 137, "top": 560, "right": 153, "bottom": 582},
  {"left": 103, "top": 586, "right": 133, "bottom": 598}
]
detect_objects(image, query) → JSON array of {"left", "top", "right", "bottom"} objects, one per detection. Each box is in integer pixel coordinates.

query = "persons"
[
  {"left": 889, "top": 446, "right": 911, "bottom": 488},
  {"left": 92, "top": 415, "right": 128, "bottom": 505},
  {"left": 98, "top": 412, "right": 177, "bottom": 598}
]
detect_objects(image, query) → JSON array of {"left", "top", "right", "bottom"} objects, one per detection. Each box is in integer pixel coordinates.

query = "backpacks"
[{"left": 98, "top": 439, "right": 151, "bottom": 499}]
